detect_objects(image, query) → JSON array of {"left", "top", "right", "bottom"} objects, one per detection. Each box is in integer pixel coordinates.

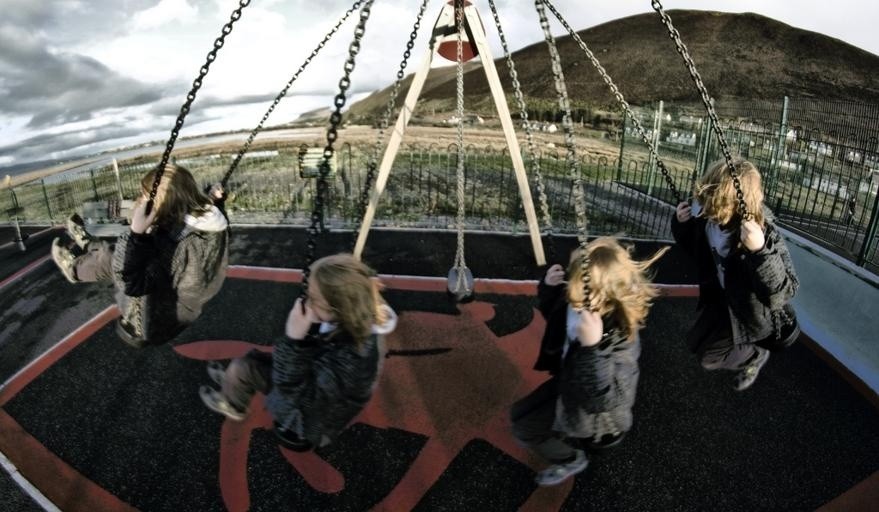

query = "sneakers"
[
  {"left": 534, "top": 449, "right": 589, "bottom": 486},
  {"left": 733, "top": 349, "right": 770, "bottom": 390},
  {"left": 199, "top": 384, "right": 245, "bottom": 422},
  {"left": 66, "top": 213, "right": 93, "bottom": 249},
  {"left": 207, "top": 362, "right": 226, "bottom": 384},
  {"left": 52, "top": 237, "right": 79, "bottom": 285}
]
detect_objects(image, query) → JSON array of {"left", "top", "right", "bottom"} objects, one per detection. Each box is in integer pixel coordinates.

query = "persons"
[
  {"left": 200, "top": 254, "right": 385, "bottom": 446},
  {"left": 671, "top": 155, "right": 800, "bottom": 391},
  {"left": 510, "top": 237, "right": 642, "bottom": 487},
  {"left": 51, "top": 163, "right": 229, "bottom": 345}
]
